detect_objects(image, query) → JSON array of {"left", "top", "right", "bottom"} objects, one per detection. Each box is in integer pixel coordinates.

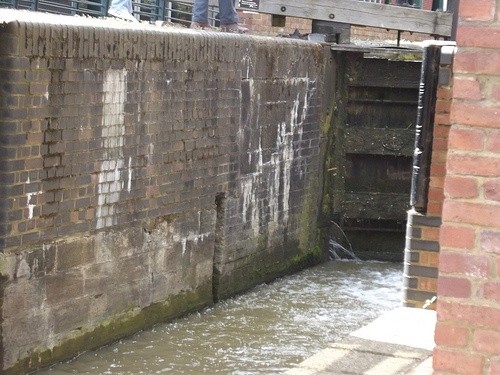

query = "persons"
[
  {"left": 190, "top": 0, "right": 249, "bottom": 34},
  {"left": 107, "top": 0, "right": 140, "bottom": 24}
]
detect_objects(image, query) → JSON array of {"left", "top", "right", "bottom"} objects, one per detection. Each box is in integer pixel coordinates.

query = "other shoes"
[
  {"left": 107, "top": 6, "right": 137, "bottom": 22},
  {"left": 221, "top": 23, "right": 249, "bottom": 34},
  {"left": 190, "top": 21, "right": 218, "bottom": 29}
]
